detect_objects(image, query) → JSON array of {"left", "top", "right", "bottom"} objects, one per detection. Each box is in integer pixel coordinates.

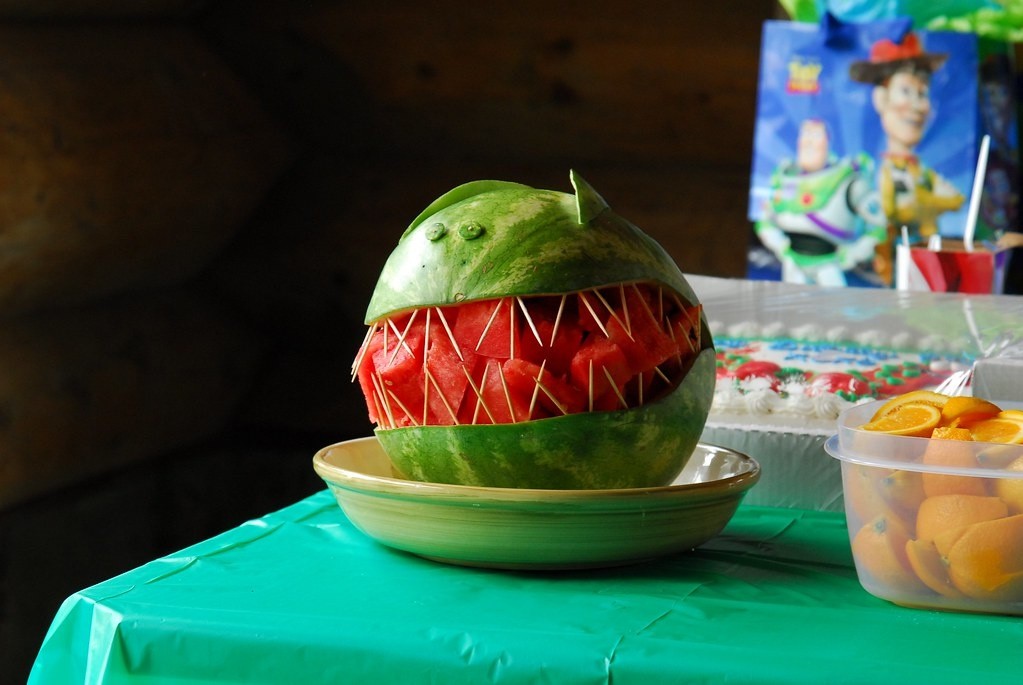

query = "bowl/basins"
[{"left": 822, "top": 399, "right": 1022, "bottom": 615}]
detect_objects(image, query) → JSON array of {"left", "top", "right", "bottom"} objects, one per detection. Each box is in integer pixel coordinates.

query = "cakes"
[{"left": 704, "top": 321, "right": 976, "bottom": 422}]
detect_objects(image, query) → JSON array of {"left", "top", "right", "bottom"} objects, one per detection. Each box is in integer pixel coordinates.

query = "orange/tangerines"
[{"left": 843, "top": 389, "right": 1023, "bottom": 605}]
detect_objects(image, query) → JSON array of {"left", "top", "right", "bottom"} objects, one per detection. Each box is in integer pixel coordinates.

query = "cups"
[{"left": 895, "top": 238, "right": 996, "bottom": 295}]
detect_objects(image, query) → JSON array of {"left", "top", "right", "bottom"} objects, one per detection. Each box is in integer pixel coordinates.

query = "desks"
[{"left": 25, "top": 487, "right": 1023, "bottom": 683}]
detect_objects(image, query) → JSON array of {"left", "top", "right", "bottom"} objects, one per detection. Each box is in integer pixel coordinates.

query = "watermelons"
[{"left": 348, "top": 170, "right": 717, "bottom": 492}]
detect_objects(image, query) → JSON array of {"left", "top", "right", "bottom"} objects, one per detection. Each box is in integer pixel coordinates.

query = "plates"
[{"left": 314, "top": 436, "right": 761, "bottom": 570}]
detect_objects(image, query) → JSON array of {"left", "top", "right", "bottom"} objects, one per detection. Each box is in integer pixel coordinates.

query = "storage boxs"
[{"left": 897, "top": 233, "right": 1023, "bottom": 295}]
